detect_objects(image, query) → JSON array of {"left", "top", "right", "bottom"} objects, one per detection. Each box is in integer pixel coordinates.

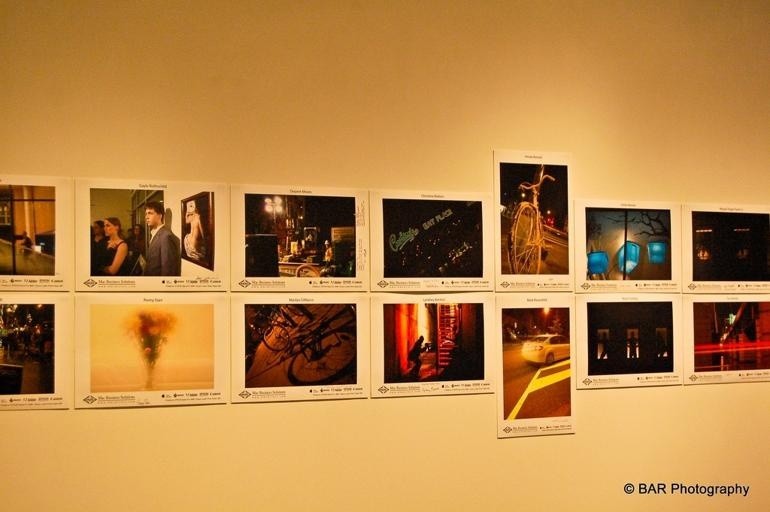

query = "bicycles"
[
  {"left": 254, "top": 304, "right": 304, "bottom": 353},
  {"left": 508, "top": 170, "right": 555, "bottom": 275}
]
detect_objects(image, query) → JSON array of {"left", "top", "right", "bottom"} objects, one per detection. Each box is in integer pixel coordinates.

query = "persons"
[
  {"left": 655, "top": 330, "right": 668, "bottom": 358},
  {"left": 143, "top": 201, "right": 180, "bottom": 276},
  {"left": 91, "top": 216, "right": 147, "bottom": 276},
  {"left": 406, "top": 336, "right": 430, "bottom": 379},
  {"left": 596, "top": 333, "right": 613, "bottom": 361},
  {"left": 624, "top": 331, "right": 641, "bottom": 359},
  {"left": 183, "top": 199, "right": 206, "bottom": 262}
]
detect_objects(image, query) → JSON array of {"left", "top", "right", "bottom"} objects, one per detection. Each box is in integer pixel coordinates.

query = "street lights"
[{"left": 264, "top": 196, "right": 283, "bottom": 223}]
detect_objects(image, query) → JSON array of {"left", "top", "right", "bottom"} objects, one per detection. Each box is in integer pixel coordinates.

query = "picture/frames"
[
  {"left": 494, "top": 149, "right": 574, "bottom": 292},
  {"left": 370, "top": 188, "right": 494, "bottom": 292},
  {"left": 574, "top": 200, "right": 681, "bottom": 293},
  {"left": 230, "top": 183, "right": 369, "bottom": 292},
  {"left": 681, "top": 202, "right": 770, "bottom": 294},
  {"left": 0, "top": 174, "right": 71, "bottom": 292},
  {"left": 494, "top": 292, "right": 576, "bottom": 440},
  {"left": 370, "top": 292, "right": 494, "bottom": 399},
  {"left": 682, "top": 295, "right": 770, "bottom": 385},
  {"left": 0, "top": 292, "right": 71, "bottom": 409},
  {"left": 74, "top": 176, "right": 228, "bottom": 292},
  {"left": 575, "top": 293, "right": 683, "bottom": 389},
  {"left": 230, "top": 292, "right": 370, "bottom": 404},
  {"left": 75, "top": 293, "right": 227, "bottom": 409}
]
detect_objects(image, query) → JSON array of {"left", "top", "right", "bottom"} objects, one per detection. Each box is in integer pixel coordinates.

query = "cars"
[{"left": 522, "top": 333, "right": 570, "bottom": 365}]
